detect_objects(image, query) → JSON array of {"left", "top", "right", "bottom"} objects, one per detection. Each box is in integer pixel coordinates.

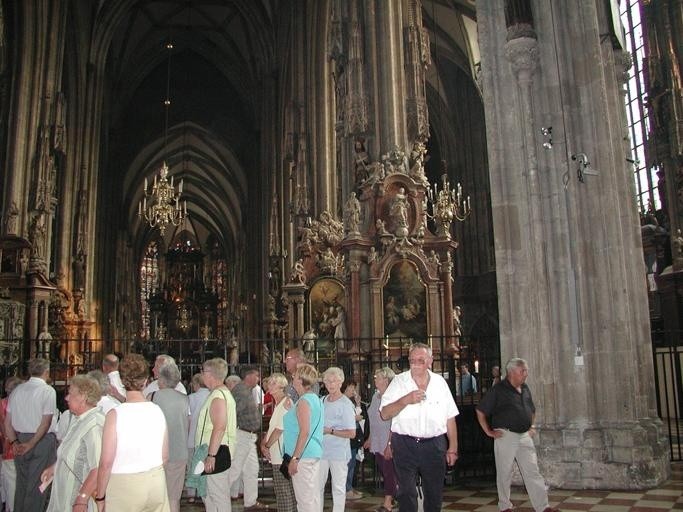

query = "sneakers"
[
  {"left": 345, "top": 489, "right": 363, "bottom": 500},
  {"left": 244, "top": 502, "right": 270, "bottom": 510}
]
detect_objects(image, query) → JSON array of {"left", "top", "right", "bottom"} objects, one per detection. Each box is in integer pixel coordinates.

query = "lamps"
[
  {"left": 540, "top": 123, "right": 555, "bottom": 149},
  {"left": 136, "top": 42, "right": 190, "bottom": 237}
]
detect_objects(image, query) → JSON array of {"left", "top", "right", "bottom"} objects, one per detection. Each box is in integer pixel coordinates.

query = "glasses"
[
  {"left": 285, "top": 355, "right": 293, "bottom": 360},
  {"left": 408, "top": 359, "right": 427, "bottom": 364},
  {"left": 200, "top": 369, "right": 209, "bottom": 376}
]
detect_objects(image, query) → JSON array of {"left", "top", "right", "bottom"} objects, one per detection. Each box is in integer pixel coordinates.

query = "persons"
[
  {"left": 457, "top": 358, "right": 560, "bottom": 511},
  {"left": 1, "top": 341, "right": 462, "bottom": 512},
  {"left": 308, "top": 210, "right": 345, "bottom": 269},
  {"left": 383, "top": 138, "right": 428, "bottom": 186},
  {"left": 341, "top": 185, "right": 412, "bottom": 235}
]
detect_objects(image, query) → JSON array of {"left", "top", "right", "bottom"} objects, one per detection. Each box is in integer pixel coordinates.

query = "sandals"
[{"left": 375, "top": 506, "right": 392, "bottom": 512}]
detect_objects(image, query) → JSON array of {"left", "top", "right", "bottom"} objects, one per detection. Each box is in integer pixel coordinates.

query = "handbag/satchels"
[
  {"left": 278, "top": 452, "right": 292, "bottom": 480},
  {"left": 200, "top": 445, "right": 232, "bottom": 476}
]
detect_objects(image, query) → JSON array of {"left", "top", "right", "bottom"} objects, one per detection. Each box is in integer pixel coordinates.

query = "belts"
[
  {"left": 407, "top": 436, "right": 435, "bottom": 443},
  {"left": 236, "top": 427, "right": 256, "bottom": 434}
]
